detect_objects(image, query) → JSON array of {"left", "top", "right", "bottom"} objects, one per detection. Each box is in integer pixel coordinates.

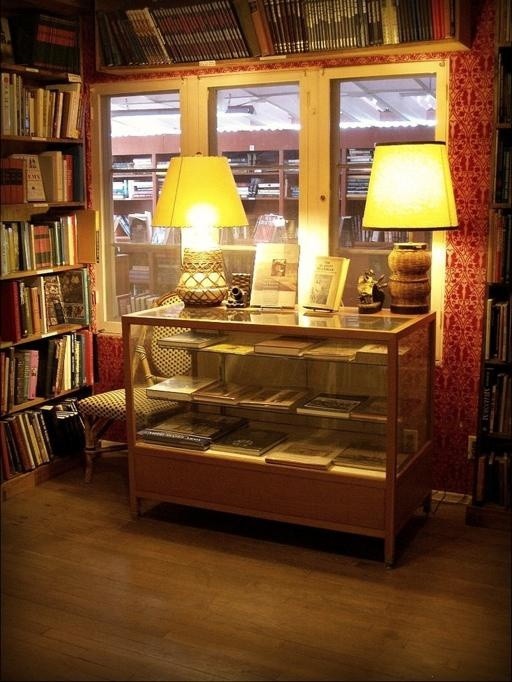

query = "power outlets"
[
  {"left": 468, "top": 436, "right": 477, "bottom": 458},
  {"left": 403, "top": 429, "right": 418, "bottom": 451}
]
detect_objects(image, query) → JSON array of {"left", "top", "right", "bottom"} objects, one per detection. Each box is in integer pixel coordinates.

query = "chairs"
[{"left": 78, "top": 289, "right": 191, "bottom": 483}]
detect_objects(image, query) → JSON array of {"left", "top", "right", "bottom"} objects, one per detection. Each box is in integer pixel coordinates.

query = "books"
[
  {"left": 1, "top": 72, "right": 88, "bottom": 140},
  {"left": 240, "top": 387, "right": 315, "bottom": 410},
  {"left": 355, "top": 342, "right": 411, "bottom": 364},
  {"left": 254, "top": 334, "right": 324, "bottom": 356},
  {"left": 146, "top": 372, "right": 219, "bottom": 402},
  {"left": 0, "top": 14, "right": 81, "bottom": 72},
  {"left": 476, "top": 445, "right": 512, "bottom": 505},
  {"left": 114, "top": 254, "right": 181, "bottom": 317},
  {"left": 301, "top": 338, "right": 364, "bottom": 360},
  {"left": 0, "top": 401, "right": 92, "bottom": 479},
  {"left": 112, "top": 209, "right": 179, "bottom": 245},
  {"left": 0, "top": 329, "right": 97, "bottom": 415},
  {"left": 1, "top": 269, "right": 96, "bottom": 342},
  {"left": 227, "top": 154, "right": 301, "bottom": 200},
  {"left": 487, "top": 209, "right": 512, "bottom": 286},
  {"left": 497, "top": 136, "right": 511, "bottom": 201},
  {"left": 351, "top": 397, "right": 420, "bottom": 421},
  {"left": 480, "top": 368, "right": 512, "bottom": 432},
  {"left": 264, "top": 435, "right": 350, "bottom": 470},
  {"left": 345, "top": 148, "right": 373, "bottom": 194},
  {"left": 498, "top": 52, "right": 511, "bottom": 125},
  {"left": 0, "top": 147, "right": 88, "bottom": 206},
  {"left": 250, "top": 243, "right": 301, "bottom": 308},
  {"left": 136, "top": 410, "right": 249, "bottom": 451},
  {"left": 157, "top": 331, "right": 228, "bottom": 348},
  {"left": 304, "top": 258, "right": 350, "bottom": 310},
  {"left": 96, "top": 1, "right": 456, "bottom": 66},
  {"left": 0, "top": 210, "right": 99, "bottom": 272},
  {"left": 209, "top": 427, "right": 289, "bottom": 456},
  {"left": 219, "top": 212, "right": 298, "bottom": 242},
  {"left": 341, "top": 214, "right": 426, "bottom": 247},
  {"left": 112, "top": 157, "right": 169, "bottom": 198},
  {"left": 192, "top": 381, "right": 263, "bottom": 404},
  {"left": 334, "top": 440, "right": 410, "bottom": 473},
  {"left": 485, "top": 297, "right": 509, "bottom": 363},
  {"left": 296, "top": 394, "right": 371, "bottom": 418}
]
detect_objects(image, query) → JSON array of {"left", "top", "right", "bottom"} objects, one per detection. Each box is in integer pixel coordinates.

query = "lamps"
[
  {"left": 361, "top": 141, "right": 459, "bottom": 315},
  {"left": 151, "top": 156, "right": 250, "bottom": 307}
]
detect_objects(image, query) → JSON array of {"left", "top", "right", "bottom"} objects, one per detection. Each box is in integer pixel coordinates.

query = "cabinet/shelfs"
[
  {"left": 122, "top": 302, "right": 437, "bottom": 570},
  {"left": 96, "top": 0, "right": 472, "bottom": 73},
  {"left": 464, "top": 46, "right": 512, "bottom": 530},
  {"left": 111, "top": 126, "right": 435, "bottom": 315},
  {"left": 0, "top": 0, "right": 96, "bottom": 502}
]
